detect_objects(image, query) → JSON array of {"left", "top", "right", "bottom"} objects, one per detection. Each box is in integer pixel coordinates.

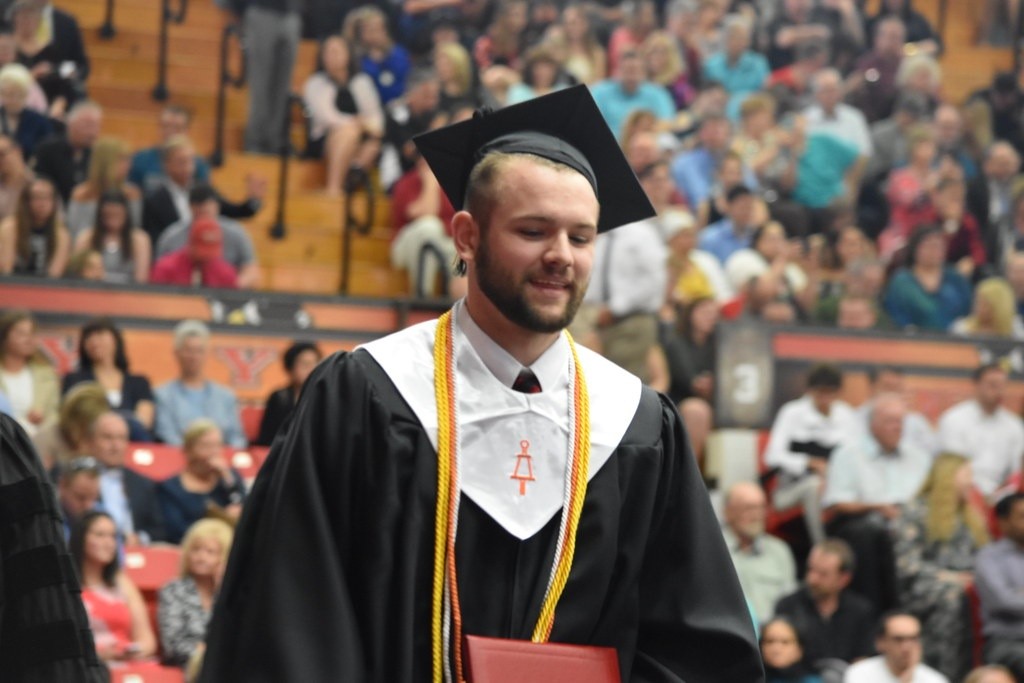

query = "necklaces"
[{"left": 433, "top": 301, "right": 590, "bottom": 683}]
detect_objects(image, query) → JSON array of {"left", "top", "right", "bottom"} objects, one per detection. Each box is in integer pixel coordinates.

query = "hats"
[
  {"left": 413, "top": 81, "right": 656, "bottom": 236},
  {"left": 189, "top": 218, "right": 225, "bottom": 260}
]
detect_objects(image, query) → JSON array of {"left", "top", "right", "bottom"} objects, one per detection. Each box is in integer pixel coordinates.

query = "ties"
[{"left": 514, "top": 368, "right": 542, "bottom": 395}]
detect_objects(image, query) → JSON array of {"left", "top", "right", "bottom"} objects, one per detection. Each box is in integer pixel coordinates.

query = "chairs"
[{"left": 127, "top": 404, "right": 270, "bottom": 650}]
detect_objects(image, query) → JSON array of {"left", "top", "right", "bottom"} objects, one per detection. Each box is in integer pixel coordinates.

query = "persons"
[
  {"left": 0, "top": 0, "right": 271, "bottom": 287},
  {"left": 225, "top": 0, "right": 1024, "bottom": 343},
  {"left": 0, "top": 411, "right": 110, "bottom": 683},
  {"left": 193, "top": 85, "right": 767, "bottom": 683},
  {"left": 0, "top": 309, "right": 322, "bottom": 683},
  {"left": 576, "top": 221, "right": 1024, "bottom": 683}
]
